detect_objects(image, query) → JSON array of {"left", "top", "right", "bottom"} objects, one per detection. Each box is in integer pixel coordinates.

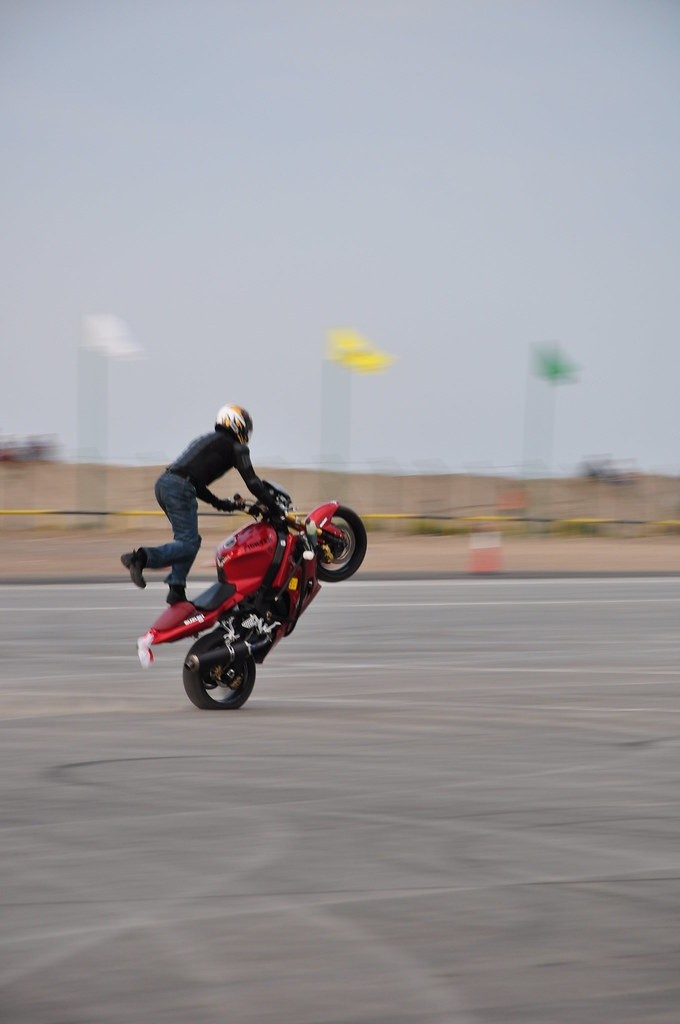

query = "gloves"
[
  {"left": 269, "top": 505, "right": 285, "bottom": 520},
  {"left": 218, "top": 498, "right": 234, "bottom": 513}
]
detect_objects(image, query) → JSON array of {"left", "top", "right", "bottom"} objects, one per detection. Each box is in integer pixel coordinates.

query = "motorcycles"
[{"left": 136, "top": 480, "right": 368, "bottom": 710}]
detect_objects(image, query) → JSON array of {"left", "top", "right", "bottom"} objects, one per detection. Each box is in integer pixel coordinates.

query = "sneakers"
[
  {"left": 120, "top": 548, "right": 146, "bottom": 588},
  {"left": 166, "top": 590, "right": 195, "bottom": 607}
]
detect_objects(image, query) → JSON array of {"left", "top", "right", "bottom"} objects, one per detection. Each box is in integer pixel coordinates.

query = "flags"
[
  {"left": 532, "top": 346, "right": 572, "bottom": 382},
  {"left": 87, "top": 315, "right": 148, "bottom": 360},
  {"left": 328, "top": 330, "right": 394, "bottom": 374}
]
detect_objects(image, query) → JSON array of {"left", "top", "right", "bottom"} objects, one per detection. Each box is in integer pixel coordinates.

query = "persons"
[{"left": 120, "top": 403, "right": 286, "bottom": 604}]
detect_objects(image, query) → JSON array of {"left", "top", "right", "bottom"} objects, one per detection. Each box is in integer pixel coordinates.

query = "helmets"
[{"left": 214, "top": 402, "right": 254, "bottom": 445}]
